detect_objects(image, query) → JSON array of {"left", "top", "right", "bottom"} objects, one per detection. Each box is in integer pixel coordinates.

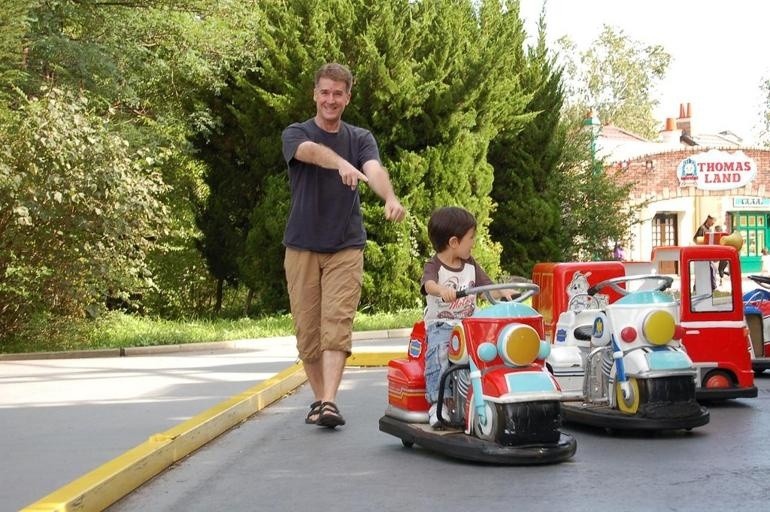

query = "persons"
[
  {"left": 613, "top": 240, "right": 624, "bottom": 259},
  {"left": 758, "top": 248, "right": 770, "bottom": 278},
  {"left": 281, "top": 64, "right": 407, "bottom": 428},
  {"left": 418, "top": 206, "right": 521, "bottom": 432},
  {"left": 714, "top": 225, "right": 725, "bottom": 233},
  {"left": 692, "top": 213, "right": 717, "bottom": 292}
]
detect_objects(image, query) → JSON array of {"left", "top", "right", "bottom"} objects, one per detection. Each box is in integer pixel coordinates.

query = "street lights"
[{"left": 581, "top": 107, "right": 603, "bottom": 232}]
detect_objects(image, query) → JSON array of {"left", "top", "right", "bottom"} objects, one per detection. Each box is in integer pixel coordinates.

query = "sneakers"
[{"left": 428, "top": 402, "right": 451, "bottom": 426}]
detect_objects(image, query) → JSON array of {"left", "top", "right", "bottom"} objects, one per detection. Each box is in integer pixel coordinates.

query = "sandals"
[
  {"left": 305, "top": 400, "right": 321, "bottom": 424},
  {"left": 319, "top": 401, "right": 344, "bottom": 426}
]
